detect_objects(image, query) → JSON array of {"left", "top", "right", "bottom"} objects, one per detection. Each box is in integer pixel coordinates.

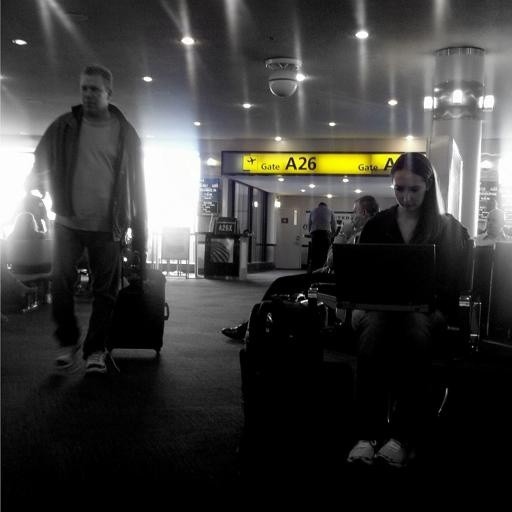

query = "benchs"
[
  {"left": 0, "top": 240, "right": 55, "bottom": 313},
  {"left": 330, "top": 242, "right": 512, "bottom": 426}
]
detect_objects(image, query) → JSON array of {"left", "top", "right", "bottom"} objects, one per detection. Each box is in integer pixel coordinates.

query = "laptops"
[{"left": 332, "top": 243, "right": 436, "bottom": 313}]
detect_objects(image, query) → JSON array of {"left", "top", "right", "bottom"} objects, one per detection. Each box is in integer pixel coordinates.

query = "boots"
[{"left": 221, "top": 323, "right": 248, "bottom": 341}]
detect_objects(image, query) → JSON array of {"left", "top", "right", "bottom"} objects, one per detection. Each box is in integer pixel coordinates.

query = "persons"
[
  {"left": 24, "top": 64, "right": 148, "bottom": 376},
  {"left": 305, "top": 202, "right": 338, "bottom": 274},
  {"left": 15, "top": 189, "right": 51, "bottom": 234},
  {"left": 6, "top": 212, "right": 53, "bottom": 307},
  {"left": 345, "top": 151, "right": 476, "bottom": 471},
  {"left": 219, "top": 196, "right": 380, "bottom": 342}
]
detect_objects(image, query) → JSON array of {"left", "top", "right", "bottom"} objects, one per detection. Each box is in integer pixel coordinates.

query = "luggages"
[
  {"left": 106, "top": 248, "right": 169, "bottom": 354},
  {"left": 238, "top": 294, "right": 308, "bottom": 434}
]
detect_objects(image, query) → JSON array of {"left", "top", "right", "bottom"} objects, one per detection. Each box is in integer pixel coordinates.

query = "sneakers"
[
  {"left": 54, "top": 339, "right": 108, "bottom": 375},
  {"left": 374, "top": 437, "right": 412, "bottom": 468},
  {"left": 347, "top": 437, "right": 377, "bottom": 465}
]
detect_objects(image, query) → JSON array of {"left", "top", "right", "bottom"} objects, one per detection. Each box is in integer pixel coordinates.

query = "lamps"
[{"left": 265, "top": 58, "right": 302, "bottom": 98}]
